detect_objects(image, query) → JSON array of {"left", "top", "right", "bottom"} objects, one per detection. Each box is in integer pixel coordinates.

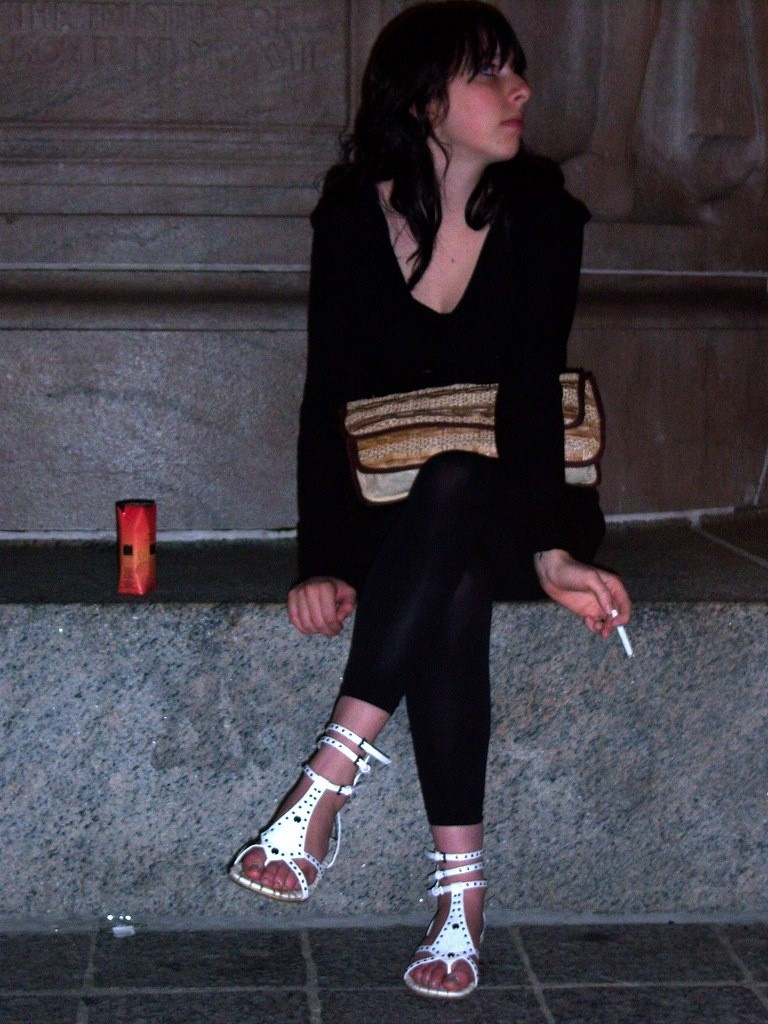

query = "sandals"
[
  {"left": 402, "top": 846, "right": 490, "bottom": 999},
  {"left": 228, "top": 723, "right": 392, "bottom": 903}
]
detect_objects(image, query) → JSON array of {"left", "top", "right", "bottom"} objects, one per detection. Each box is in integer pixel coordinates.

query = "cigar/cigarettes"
[{"left": 611, "top": 609, "right": 635, "bottom": 661}]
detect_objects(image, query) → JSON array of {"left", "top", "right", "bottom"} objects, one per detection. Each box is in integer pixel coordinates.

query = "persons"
[{"left": 227, "top": 1, "right": 632, "bottom": 998}]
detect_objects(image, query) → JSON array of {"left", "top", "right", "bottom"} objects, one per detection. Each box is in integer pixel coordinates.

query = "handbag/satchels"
[{"left": 345, "top": 372, "right": 606, "bottom": 505}]
